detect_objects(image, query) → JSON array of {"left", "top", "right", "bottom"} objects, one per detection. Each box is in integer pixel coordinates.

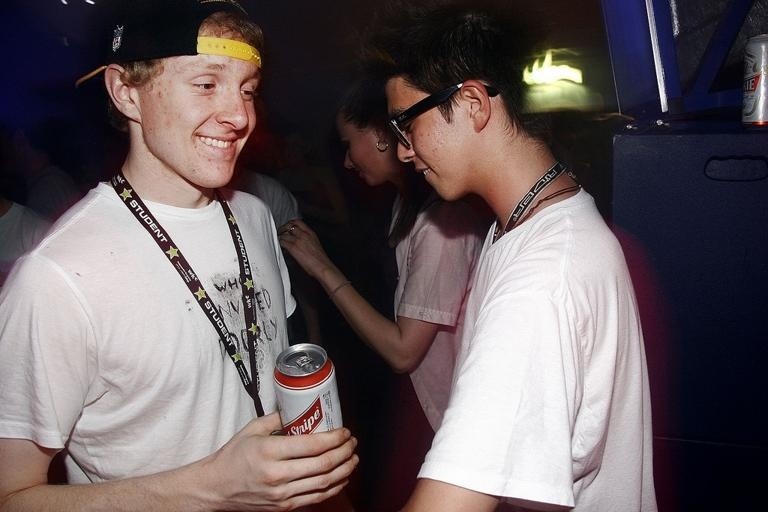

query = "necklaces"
[{"left": 491, "top": 182, "right": 581, "bottom": 246}]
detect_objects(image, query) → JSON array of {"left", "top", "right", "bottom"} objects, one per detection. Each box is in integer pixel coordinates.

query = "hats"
[{"left": 74, "top": 1, "right": 262, "bottom": 96}]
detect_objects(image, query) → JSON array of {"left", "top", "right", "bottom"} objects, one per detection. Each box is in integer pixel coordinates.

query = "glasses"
[{"left": 389, "top": 82, "right": 499, "bottom": 150}]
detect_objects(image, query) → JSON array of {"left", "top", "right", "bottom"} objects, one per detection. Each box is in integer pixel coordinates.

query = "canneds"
[
  {"left": 741, "top": 33, "right": 768, "bottom": 130},
  {"left": 273, "top": 343, "right": 345, "bottom": 459}
]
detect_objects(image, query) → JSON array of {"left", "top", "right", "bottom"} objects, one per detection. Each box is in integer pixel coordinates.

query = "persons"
[
  {"left": 0, "top": 1, "right": 365, "bottom": 512},
  {"left": 0, "top": 110, "right": 89, "bottom": 285},
  {"left": 269, "top": 78, "right": 494, "bottom": 452},
  {"left": 220, "top": 104, "right": 356, "bottom": 350},
  {"left": 375, "top": 8, "right": 666, "bottom": 510}
]
